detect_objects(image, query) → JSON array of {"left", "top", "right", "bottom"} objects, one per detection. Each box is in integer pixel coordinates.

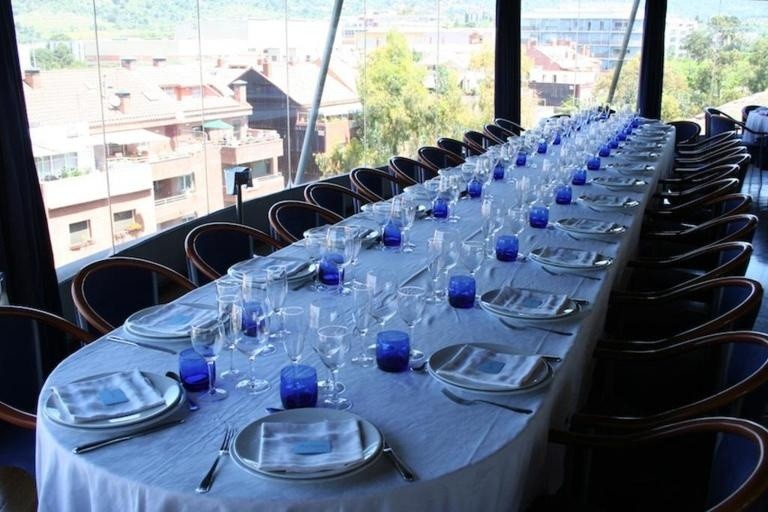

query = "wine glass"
[{"left": 190, "top": 103, "right": 639, "bottom": 412}]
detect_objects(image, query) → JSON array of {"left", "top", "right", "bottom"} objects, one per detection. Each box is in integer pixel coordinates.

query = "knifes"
[
  {"left": 72, "top": 402, "right": 192, "bottom": 455},
  {"left": 380, "top": 434, "right": 418, "bottom": 483},
  {"left": 107, "top": 334, "right": 178, "bottom": 357}
]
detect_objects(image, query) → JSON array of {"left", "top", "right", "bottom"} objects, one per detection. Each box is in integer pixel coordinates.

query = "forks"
[
  {"left": 441, "top": 388, "right": 534, "bottom": 417},
  {"left": 497, "top": 315, "right": 573, "bottom": 340},
  {"left": 196, "top": 425, "right": 239, "bottom": 494},
  {"left": 541, "top": 266, "right": 601, "bottom": 283},
  {"left": 566, "top": 231, "right": 616, "bottom": 246}
]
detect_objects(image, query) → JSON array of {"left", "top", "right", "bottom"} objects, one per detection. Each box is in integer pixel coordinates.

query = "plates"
[
  {"left": 48, "top": 372, "right": 187, "bottom": 430},
  {"left": 481, "top": 288, "right": 583, "bottom": 324},
  {"left": 228, "top": 257, "right": 319, "bottom": 287},
  {"left": 424, "top": 341, "right": 554, "bottom": 399},
  {"left": 555, "top": 218, "right": 627, "bottom": 243},
  {"left": 594, "top": 177, "right": 647, "bottom": 191},
  {"left": 230, "top": 409, "right": 387, "bottom": 482},
  {"left": 123, "top": 303, "right": 230, "bottom": 341},
  {"left": 529, "top": 246, "right": 616, "bottom": 274},
  {"left": 638, "top": 122, "right": 675, "bottom": 186},
  {"left": 581, "top": 194, "right": 639, "bottom": 215}
]
